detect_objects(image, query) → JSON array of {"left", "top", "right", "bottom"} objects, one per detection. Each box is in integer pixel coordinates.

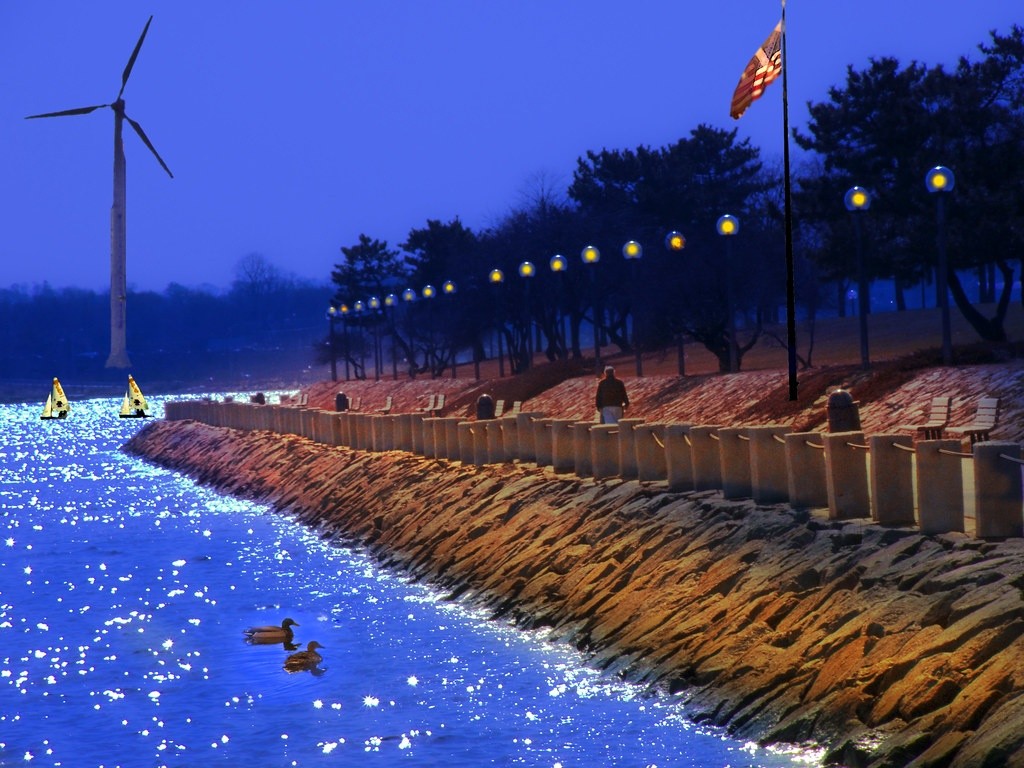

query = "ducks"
[
  {"left": 243, "top": 618, "right": 300, "bottom": 639},
  {"left": 284, "top": 641, "right": 324, "bottom": 665}
]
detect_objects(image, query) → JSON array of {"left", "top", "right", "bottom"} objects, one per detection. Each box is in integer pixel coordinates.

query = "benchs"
[
  {"left": 900, "top": 397, "right": 951, "bottom": 440},
  {"left": 288, "top": 394, "right": 303, "bottom": 408},
  {"left": 351, "top": 397, "right": 361, "bottom": 411},
  {"left": 495, "top": 400, "right": 505, "bottom": 417},
  {"left": 513, "top": 401, "right": 522, "bottom": 415},
  {"left": 374, "top": 397, "right": 392, "bottom": 414},
  {"left": 416, "top": 395, "right": 436, "bottom": 417},
  {"left": 944, "top": 397, "right": 1000, "bottom": 453},
  {"left": 297, "top": 394, "right": 308, "bottom": 407},
  {"left": 432, "top": 395, "right": 445, "bottom": 417}
]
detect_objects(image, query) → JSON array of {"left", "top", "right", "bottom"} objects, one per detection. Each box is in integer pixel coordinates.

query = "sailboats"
[
  {"left": 118, "top": 374, "right": 156, "bottom": 418},
  {"left": 39, "top": 376, "right": 71, "bottom": 420}
]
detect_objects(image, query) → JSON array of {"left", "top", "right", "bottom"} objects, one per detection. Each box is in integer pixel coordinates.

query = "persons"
[
  {"left": 135, "top": 408, "right": 145, "bottom": 415},
  {"left": 595, "top": 366, "right": 630, "bottom": 424},
  {"left": 59, "top": 410, "right": 67, "bottom": 418}
]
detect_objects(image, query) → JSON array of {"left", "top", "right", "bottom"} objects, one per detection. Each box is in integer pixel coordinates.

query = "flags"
[{"left": 729, "top": 20, "right": 783, "bottom": 119}]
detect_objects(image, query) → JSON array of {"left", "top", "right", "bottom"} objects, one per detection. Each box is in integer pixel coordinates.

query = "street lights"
[
  {"left": 354, "top": 300, "right": 368, "bottom": 379},
  {"left": 403, "top": 288, "right": 417, "bottom": 379},
  {"left": 717, "top": 214, "right": 743, "bottom": 372},
  {"left": 581, "top": 245, "right": 602, "bottom": 376},
  {"left": 422, "top": 284, "right": 437, "bottom": 379},
  {"left": 327, "top": 306, "right": 338, "bottom": 381},
  {"left": 665, "top": 229, "right": 686, "bottom": 375},
  {"left": 368, "top": 296, "right": 381, "bottom": 380},
  {"left": 517, "top": 260, "right": 537, "bottom": 367},
  {"left": 927, "top": 165, "right": 962, "bottom": 368},
  {"left": 490, "top": 269, "right": 506, "bottom": 379},
  {"left": 620, "top": 241, "right": 646, "bottom": 376},
  {"left": 549, "top": 255, "right": 571, "bottom": 360},
  {"left": 339, "top": 303, "right": 350, "bottom": 380},
  {"left": 442, "top": 280, "right": 457, "bottom": 378},
  {"left": 845, "top": 186, "right": 874, "bottom": 372},
  {"left": 385, "top": 293, "right": 400, "bottom": 380}
]
